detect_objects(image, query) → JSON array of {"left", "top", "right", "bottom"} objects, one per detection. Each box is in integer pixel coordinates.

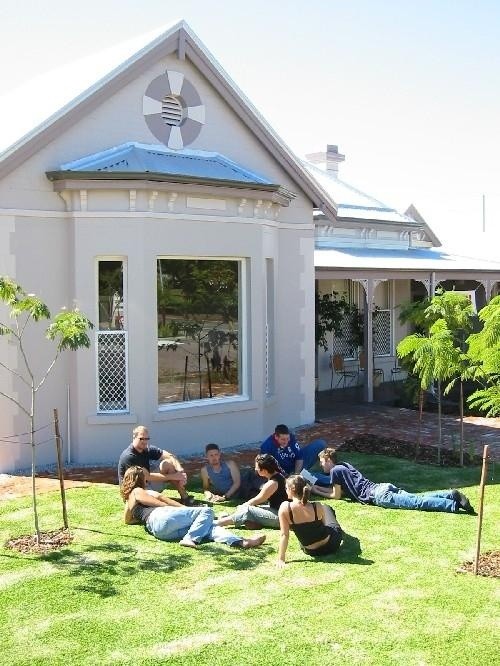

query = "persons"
[
  {"left": 304, "top": 447, "right": 477, "bottom": 521},
  {"left": 276, "top": 474, "right": 341, "bottom": 562},
  {"left": 122, "top": 466, "right": 268, "bottom": 549},
  {"left": 201, "top": 441, "right": 266, "bottom": 503},
  {"left": 261, "top": 425, "right": 335, "bottom": 487},
  {"left": 212, "top": 455, "right": 294, "bottom": 528},
  {"left": 118, "top": 426, "right": 200, "bottom": 507}
]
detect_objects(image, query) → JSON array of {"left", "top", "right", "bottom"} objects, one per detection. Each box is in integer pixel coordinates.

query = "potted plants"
[
  {"left": 350, "top": 299, "right": 382, "bottom": 352},
  {"left": 316, "top": 287, "right": 358, "bottom": 392}
]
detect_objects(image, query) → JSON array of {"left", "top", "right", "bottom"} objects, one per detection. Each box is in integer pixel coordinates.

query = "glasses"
[{"left": 138, "top": 437, "right": 150, "bottom": 441}]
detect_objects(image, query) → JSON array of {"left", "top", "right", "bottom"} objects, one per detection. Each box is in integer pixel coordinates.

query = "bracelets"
[{"left": 223, "top": 494, "right": 229, "bottom": 500}]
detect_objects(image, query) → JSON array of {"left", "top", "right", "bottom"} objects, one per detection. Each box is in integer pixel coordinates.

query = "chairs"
[{"left": 330, "top": 352, "right": 413, "bottom": 394}]
